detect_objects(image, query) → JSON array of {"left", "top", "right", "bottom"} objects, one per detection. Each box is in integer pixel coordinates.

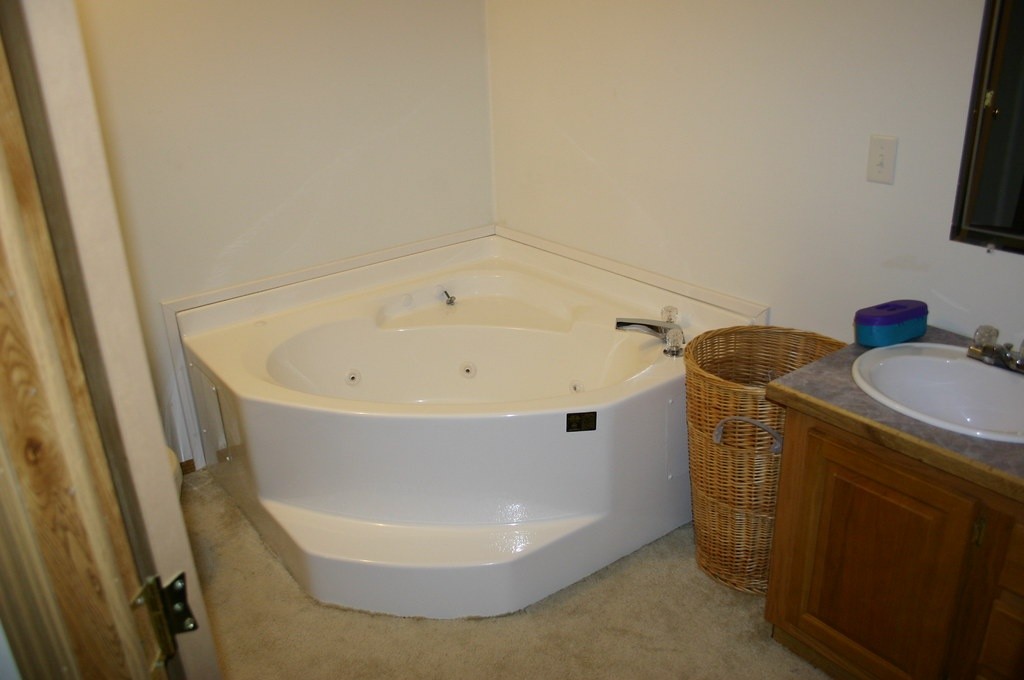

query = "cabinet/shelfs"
[{"left": 763, "top": 406, "right": 1024, "bottom": 680}]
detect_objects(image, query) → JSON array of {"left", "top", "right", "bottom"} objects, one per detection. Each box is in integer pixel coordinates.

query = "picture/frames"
[{"left": 949, "top": 0, "right": 1024, "bottom": 256}]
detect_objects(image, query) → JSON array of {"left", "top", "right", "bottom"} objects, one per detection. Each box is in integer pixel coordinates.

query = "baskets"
[{"left": 684, "top": 325, "right": 848, "bottom": 598}]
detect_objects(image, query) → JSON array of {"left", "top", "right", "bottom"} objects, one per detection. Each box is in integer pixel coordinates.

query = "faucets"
[
  {"left": 615, "top": 316, "right": 683, "bottom": 340},
  {"left": 967, "top": 323, "right": 1024, "bottom": 381}
]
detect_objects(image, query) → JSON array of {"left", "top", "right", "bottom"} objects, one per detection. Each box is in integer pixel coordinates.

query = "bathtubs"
[{"left": 183, "top": 258, "right": 730, "bottom": 619}]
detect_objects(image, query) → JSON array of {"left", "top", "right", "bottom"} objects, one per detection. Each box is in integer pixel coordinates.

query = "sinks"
[{"left": 851, "top": 341, "right": 1024, "bottom": 448}]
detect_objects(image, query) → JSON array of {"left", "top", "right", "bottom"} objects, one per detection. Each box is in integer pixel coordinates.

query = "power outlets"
[{"left": 866, "top": 135, "right": 898, "bottom": 185}]
